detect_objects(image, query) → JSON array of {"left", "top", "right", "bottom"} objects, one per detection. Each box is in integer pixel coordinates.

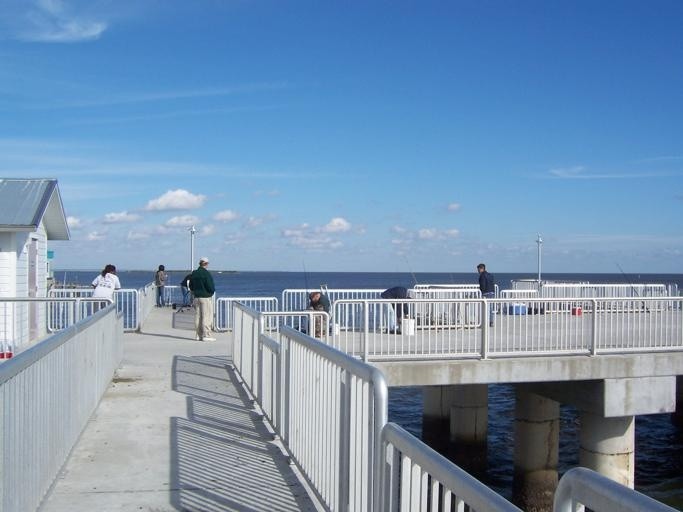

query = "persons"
[
  {"left": 155, "top": 265, "right": 167, "bottom": 306},
  {"left": 476, "top": 263, "right": 494, "bottom": 325},
  {"left": 300, "top": 292, "right": 331, "bottom": 336},
  {"left": 91, "top": 265, "right": 121, "bottom": 312},
  {"left": 381, "top": 287, "right": 411, "bottom": 333},
  {"left": 180, "top": 270, "right": 195, "bottom": 306},
  {"left": 191, "top": 258, "right": 217, "bottom": 341}
]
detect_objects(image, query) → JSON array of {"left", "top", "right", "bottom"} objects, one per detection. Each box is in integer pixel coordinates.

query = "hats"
[{"left": 200, "top": 257, "right": 209, "bottom": 263}]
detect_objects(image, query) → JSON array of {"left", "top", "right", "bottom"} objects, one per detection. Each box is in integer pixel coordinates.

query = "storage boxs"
[{"left": 502, "top": 301, "right": 528, "bottom": 317}]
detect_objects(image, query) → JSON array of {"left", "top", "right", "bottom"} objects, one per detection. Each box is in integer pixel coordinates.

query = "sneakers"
[{"left": 197, "top": 337, "right": 216, "bottom": 343}]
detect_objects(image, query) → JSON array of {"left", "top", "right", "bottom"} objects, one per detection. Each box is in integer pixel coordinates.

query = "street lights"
[
  {"left": 535, "top": 234, "right": 542, "bottom": 287},
  {"left": 190, "top": 225, "right": 196, "bottom": 272}
]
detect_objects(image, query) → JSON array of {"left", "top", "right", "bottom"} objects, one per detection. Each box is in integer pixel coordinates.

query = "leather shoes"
[
  {"left": 386, "top": 328, "right": 388, "bottom": 333},
  {"left": 390, "top": 330, "right": 400, "bottom": 333}
]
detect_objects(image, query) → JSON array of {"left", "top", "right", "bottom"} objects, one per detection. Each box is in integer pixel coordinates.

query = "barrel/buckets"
[
  {"left": 329, "top": 323, "right": 340, "bottom": 336},
  {"left": 400, "top": 319, "right": 415, "bottom": 336}
]
